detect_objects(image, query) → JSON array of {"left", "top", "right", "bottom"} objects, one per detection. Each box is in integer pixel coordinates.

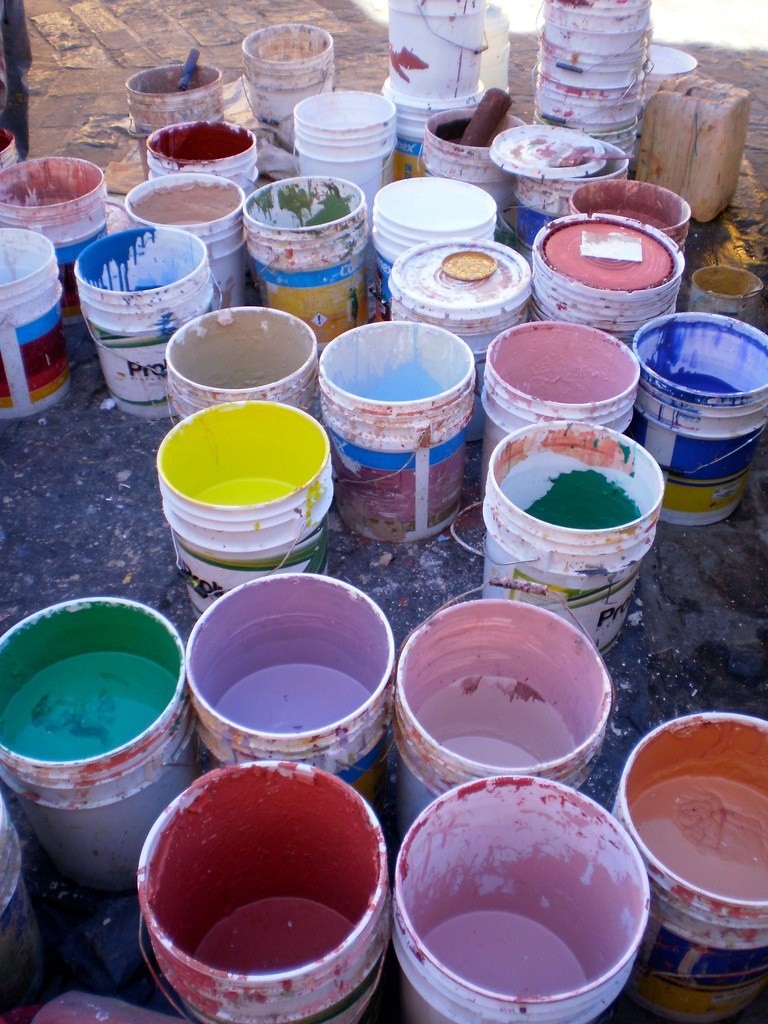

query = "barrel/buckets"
[{"left": 2, "top": 1, "right": 767, "bottom": 1024}]
